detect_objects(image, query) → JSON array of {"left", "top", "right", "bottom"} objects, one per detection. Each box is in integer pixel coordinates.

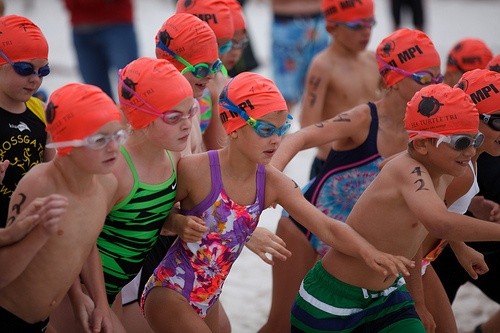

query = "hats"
[
  {"left": 446, "top": 39, "right": 492, "bottom": 72},
  {"left": 218, "top": 71, "right": 288, "bottom": 136},
  {"left": 376, "top": 29, "right": 441, "bottom": 87},
  {"left": 46, "top": 85, "right": 123, "bottom": 156},
  {"left": 452, "top": 67, "right": 500, "bottom": 115},
  {"left": 176, "top": 0, "right": 234, "bottom": 40},
  {"left": 0, "top": 15, "right": 49, "bottom": 67},
  {"left": 155, "top": 12, "right": 218, "bottom": 72},
  {"left": 321, "top": 0, "right": 374, "bottom": 22},
  {"left": 403, "top": 83, "right": 479, "bottom": 139},
  {"left": 117, "top": 58, "right": 194, "bottom": 129},
  {"left": 223, "top": 0, "right": 245, "bottom": 32},
  {"left": 485, "top": 52, "right": 500, "bottom": 73}
]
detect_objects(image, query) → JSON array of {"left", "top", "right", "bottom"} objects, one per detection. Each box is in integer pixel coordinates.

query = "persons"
[
  {"left": 390, "top": 0, "right": 425, "bottom": 34},
  {"left": 1, "top": 0, "right": 500, "bottom": 333},
  {"left": 63, "top": 0, "right": 138, "bottom": 103},
  {"left": 272, "top": 0, "right": 331, "bottom": 116}
]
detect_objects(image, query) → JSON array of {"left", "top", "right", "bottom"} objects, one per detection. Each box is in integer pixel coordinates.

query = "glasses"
[
  {"left": 254, "top": 116, "right": 291, "bottom": 138},
  {"left": 216, "top": 41, "right": 233, "bottom": 54},
  {"left": 482, "top": 114, "right": 500, "bottom": 132},
  {"left": 162, "top": 106, "right": 200, "bottom": 126},
  {"left": 233, "top": 38, "right": 248, "bottom": 50},
  {"left": 45, "top": 132, "right": 127, "bottom": 149},
  {"left": 192, "top": 58, "right": 223, "bottom": 80},
  {"left": 449, "top": 132, "right": 485, "bottom": 151},
  {"left": 343, "top": 19, "right": 375, "bottom": 30},
  {"left": 10, "top": 62, "right": 51, "bottom": 76},
  {"left": 410, "top": 71, "right": 444, "bottom": 85}
]
